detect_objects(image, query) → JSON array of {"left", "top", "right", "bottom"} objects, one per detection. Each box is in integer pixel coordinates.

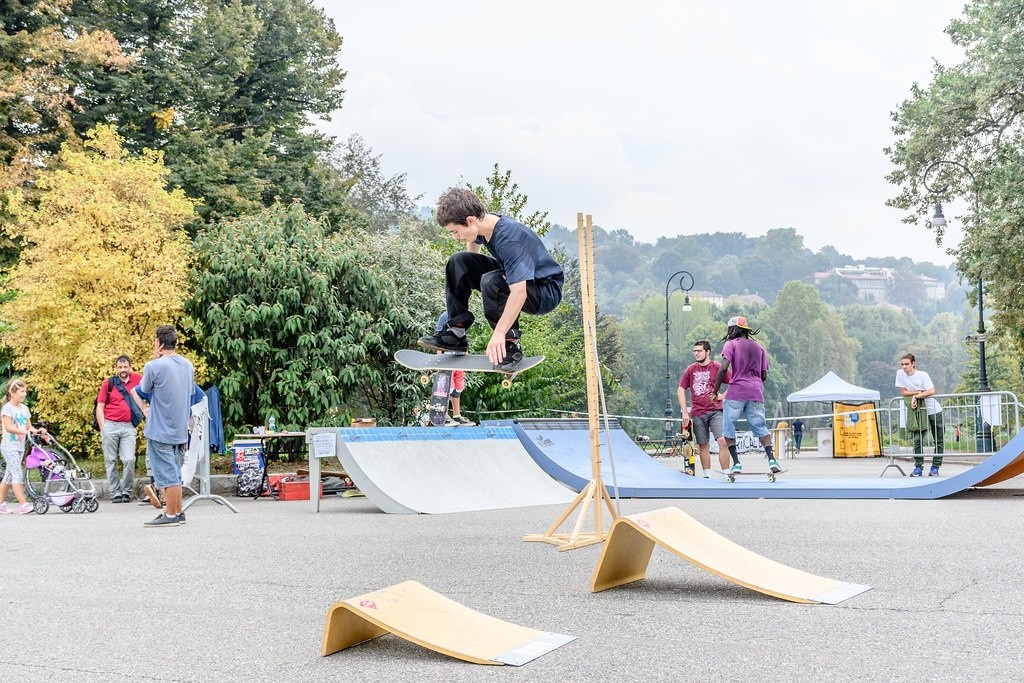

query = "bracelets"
[
  {"left": 713, "top": 391, "right": 719, "bottom": 395},
  {"left": 914, "top": 395, "right": 917, "bottom": 399}
]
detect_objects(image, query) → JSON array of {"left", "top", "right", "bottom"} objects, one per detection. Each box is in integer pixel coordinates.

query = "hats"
[{"left": 728, "top": 316, "right": 752, "bottom": 329}]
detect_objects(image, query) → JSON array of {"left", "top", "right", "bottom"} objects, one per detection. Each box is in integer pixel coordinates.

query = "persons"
[
  {"left": 777, "top": 420, "right": 788, "bottom": 441},
  {"left": 141, "top": 324, "right": 195, "bottom": 526},
  {"left": 130, "top": 382, "right": 205, "bottom": 508},
  {"left": 956, "top": 424, "right": 963, "bottom": 442},
  {"left": 676, "top": 341, "right": 732, "bottom": 477},
  {"left": 0, "top": 379, "right": 47, "bottom": 514},
  {"left": 710, "top": 317, "right": 782, "bottom": 472},
  {"left": 789, "top": 419, "right": 804, "bottom": 453},
  {"left": 95, "top": 356, "right": 142, "bottom": 503},
  {"left": 436, "top": 310, "right": 476, "bottom": 426},
  {"left": 895, "top": 353, "right": 945, "bottom": 477},
  {"left": 418, "top": 187, "right": 564, "bottom": 372}
]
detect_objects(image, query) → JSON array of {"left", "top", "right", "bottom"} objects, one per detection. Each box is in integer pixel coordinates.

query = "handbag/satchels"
[
  {"left": 235, "top": 467, "right": 268, "bottom": 498},
  {"left": 125, "top": 393, "right": 145, "bottom": 428}
]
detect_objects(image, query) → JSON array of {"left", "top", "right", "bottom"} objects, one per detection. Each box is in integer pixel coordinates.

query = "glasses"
[{"left": 692, "top": 348, "right": 704, "bottom": 352}]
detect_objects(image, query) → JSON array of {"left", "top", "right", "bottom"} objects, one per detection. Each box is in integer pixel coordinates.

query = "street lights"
[
  {"left": 665, "top": 270, "right": 695, "bottom": 448},
  {"left": 923, "top": 159, "right": 997, "bottom": 454}
]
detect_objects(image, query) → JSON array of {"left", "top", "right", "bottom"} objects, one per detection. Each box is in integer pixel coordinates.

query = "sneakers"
[
  {"left": 453, "top": 416, "right": 476, "bottom": 427},
  {"left": 0, "top": 502, "right": 14, "bottom": 514},
  {"left": 417, "top": 325, "right": 469, "bottom": 355},
  {"left": 910, "top": 467, "right": 922, "bottom": 476},
  {"left": 494, "top": 340, "right": 524, "bottom": 373},
  {"left": 929, "top": 466, "right": 939, "bottom": 476},
  {"left": 731, "top": 463, "right": 742, "bottom": 473},
  {"left": 179, "top": 513, "right": 186, "bottom": 524},
  {"left": 769, "top": 460, "right": 781, "bottom": 472},
  {"left": 20, "top": 502, "right": 34, "bottom": 514},
  {"left": 144, "top": 511, "right": 180, "bottom": 527},
  {"left": 444, "top": 415, "right": 460, "bottom": 427}
]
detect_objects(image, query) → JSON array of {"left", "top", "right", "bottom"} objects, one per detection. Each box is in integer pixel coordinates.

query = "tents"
[{"left": 787, "top": 371, "right": 884, "bottom": 457}]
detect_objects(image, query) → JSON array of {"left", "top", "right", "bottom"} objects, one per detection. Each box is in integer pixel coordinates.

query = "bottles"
[{"left": 269, "top": 416, "right": 275, "bottom": 433}]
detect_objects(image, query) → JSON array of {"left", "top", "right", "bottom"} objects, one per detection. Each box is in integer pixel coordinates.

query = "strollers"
[{"left": 24, "top": 430, "right": 99, "bottom": 516}]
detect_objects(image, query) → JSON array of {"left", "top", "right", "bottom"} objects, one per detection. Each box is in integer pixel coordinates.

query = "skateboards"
[
  {"left": 716, "top": 469, "right": 788, "bottom": 483},
  {"left": 393, "top": 350, "right": 546, "bottom": 389},
  {"left": 675, "top": 419, "right": 695, "bottom": 477},
  {"left": 425, "top": 370, "right": 452, "bottom": 427}
]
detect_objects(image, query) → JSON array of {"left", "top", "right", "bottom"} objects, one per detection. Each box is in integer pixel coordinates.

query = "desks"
[
  {"left": 634, "top": 438, "right": 683, "bottom": 459},
  {"left": 767, "top": 427, "right": 792, "bottom": 460},
  {"left": 233, "top": 429, "right": 307, "bottom": 501},
  {"left": 810, "top": 426, "right": 833, "bottom": 458}
]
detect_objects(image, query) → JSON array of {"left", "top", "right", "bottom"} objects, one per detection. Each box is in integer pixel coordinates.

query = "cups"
[{"left": 259, "top": 426, "right": 265, "bottom": 435}]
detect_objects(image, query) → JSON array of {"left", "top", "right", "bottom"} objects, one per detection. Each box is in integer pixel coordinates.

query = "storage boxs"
[
  {"left": 350, "top": 416, "right": 377, "bottom": 428},
  {"left": 277, "top": 479, "right": 324, "bottom": 500},
  {"left": 260, "top": 473, "right": 299, "bottom": 498}
]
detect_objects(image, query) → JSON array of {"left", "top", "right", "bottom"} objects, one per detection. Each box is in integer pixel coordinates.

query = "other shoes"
[
  {"left": 138, "top": 497, "right": 151, "bottom": 507},
  {"left": 112, "top": 497, "right": 122, "bottom": 503},
  {"left": 123, "top": 494, "right": 131, "bottom": 502},
  {"left": 145, "top": 484, "right": 163, "bottom": 509}
]
